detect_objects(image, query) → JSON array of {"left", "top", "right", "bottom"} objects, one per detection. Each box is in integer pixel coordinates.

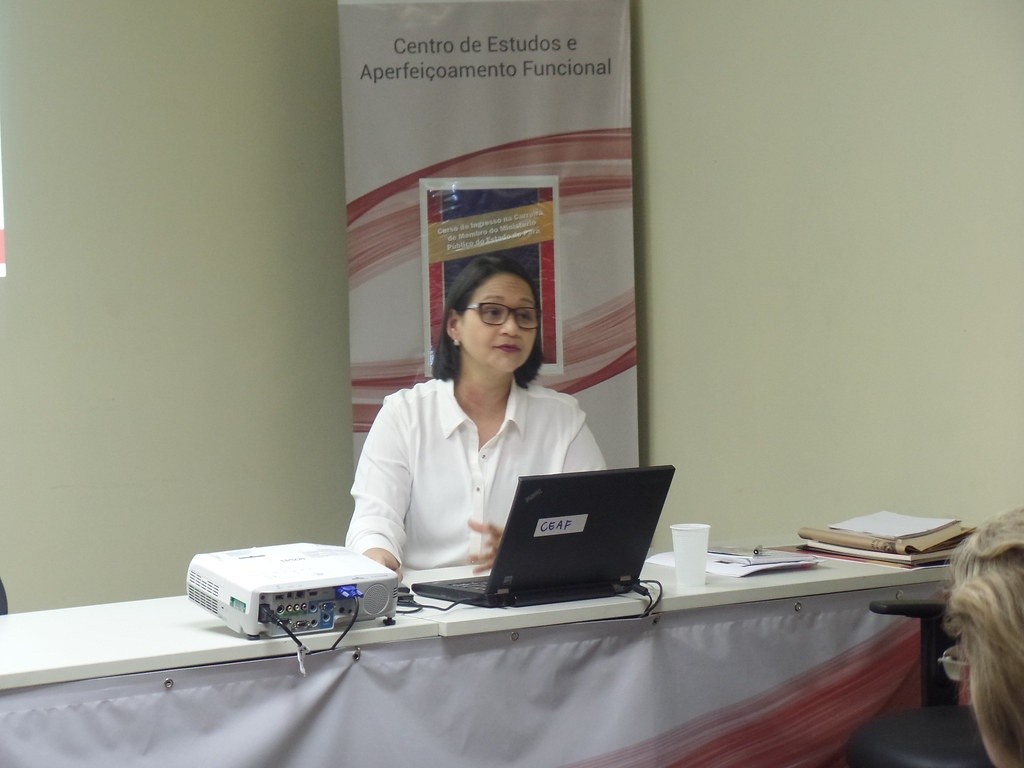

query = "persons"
[
  {"left": 343, "top": 253, "right": 607, "bottom": 584},
  {"left": 939, "top": 501, "right": 1023, "bottom": 768}
]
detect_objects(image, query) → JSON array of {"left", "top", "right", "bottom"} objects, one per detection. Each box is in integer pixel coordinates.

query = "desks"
[{"left": 0, "top": 547, "right": 961, "bottom": 710}]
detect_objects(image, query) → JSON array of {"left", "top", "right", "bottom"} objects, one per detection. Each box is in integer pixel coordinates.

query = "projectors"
[{"left": 184, "top": 543, "right": 399, "bottom": 636}]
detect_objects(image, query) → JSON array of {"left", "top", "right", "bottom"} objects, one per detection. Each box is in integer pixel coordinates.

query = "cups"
[{"left": 670, "top": 524, "right": 711, "bottom": 585}]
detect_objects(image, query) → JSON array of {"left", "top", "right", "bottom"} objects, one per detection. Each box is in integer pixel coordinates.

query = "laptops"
[{"left": 411, "top": 466, "right": 676, "bottom": 607}]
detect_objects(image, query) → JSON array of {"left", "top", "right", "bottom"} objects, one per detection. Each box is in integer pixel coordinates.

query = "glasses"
[
  {"left": 461, "top": 302, "right": 540, "bottom": 330},
  {"left": 937, "top": 644, "right": 971, "bottom": 680}
]
